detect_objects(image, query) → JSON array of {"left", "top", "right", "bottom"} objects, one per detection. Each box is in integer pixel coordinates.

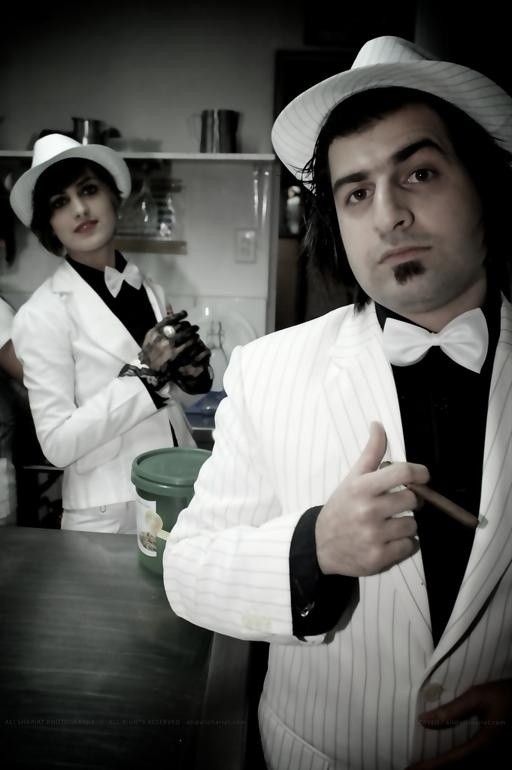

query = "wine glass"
[{"left": 160, "top": 323, "right": 177, "bottom": 338}]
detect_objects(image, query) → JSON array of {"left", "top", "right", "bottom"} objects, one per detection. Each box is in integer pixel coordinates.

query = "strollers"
[
  {"left": 103, "top": 259, "right": 142, "bottom": 297},
  {"left": 379, "top": 306, "right": 489, "bottom": 376}
]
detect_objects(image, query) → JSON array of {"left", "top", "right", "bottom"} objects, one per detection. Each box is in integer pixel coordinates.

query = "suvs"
[{"left": 130, "top": 447, "right": 213, "bottom": 577}]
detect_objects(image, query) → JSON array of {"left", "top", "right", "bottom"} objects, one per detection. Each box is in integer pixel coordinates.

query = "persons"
[
  {"left": 155, "top": 27, "right": 512, "bottom": 769},
  {"left": 8, "top": 132, "right": 214, "bottom": 534},
  {"left": 0, "top": 186, "right": 37, "bottom": 525}
]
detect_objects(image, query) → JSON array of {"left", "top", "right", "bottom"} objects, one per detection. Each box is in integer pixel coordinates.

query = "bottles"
[
  {"left": 10, "top": 133, "right": 132, "bottom": 229},
  {"left": 271, "top": 36, "right": 511, "bottom": 200}
]
